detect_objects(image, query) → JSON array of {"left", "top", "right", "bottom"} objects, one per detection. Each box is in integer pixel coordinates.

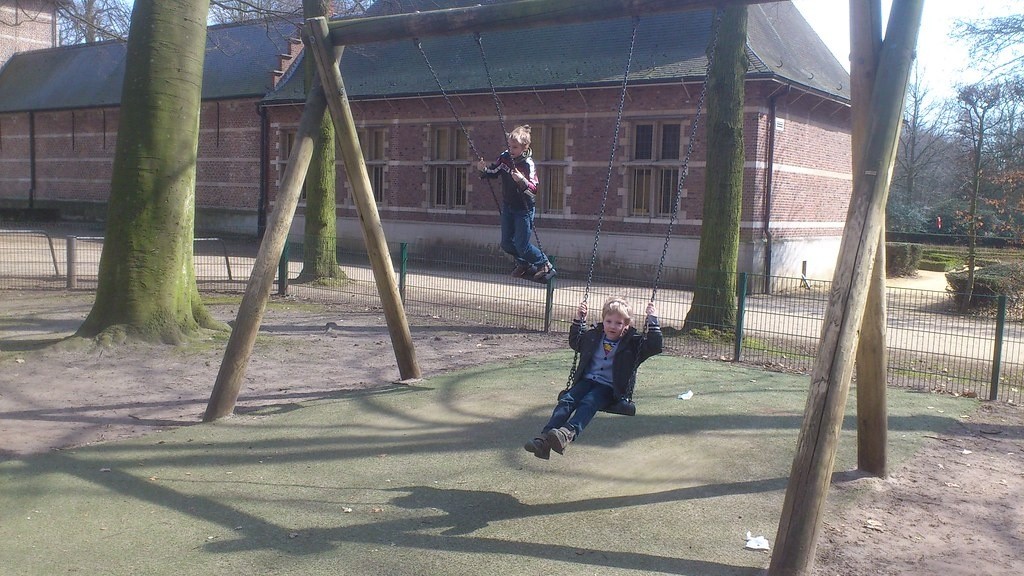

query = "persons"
[
  {"left": 477, "top": 124, "right": 552, "bottom": 281},
  {"left": 524, "top": 301, "right": 664, "bottom": 460}
]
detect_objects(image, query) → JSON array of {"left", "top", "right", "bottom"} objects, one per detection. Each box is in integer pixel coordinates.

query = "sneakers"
[
  {"left": 534, "top": 262, "right": 553, "bottom": 279},
  {"left": 546, "top": 423, "right": 576, "bottom": 455},
  {"left": 524, "top": 433, "right": 551, "bottom": 460},
  {"left": 510, "top": 261, "right": 533, "bottom": 277}
]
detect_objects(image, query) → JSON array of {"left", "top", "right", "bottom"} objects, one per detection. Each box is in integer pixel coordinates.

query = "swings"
[
  {"left": 413, "top": 32, "right": 556, "bottom": 284},
  {"left": 553, "top": 7, "right": 722, "bottom": 417}
]
